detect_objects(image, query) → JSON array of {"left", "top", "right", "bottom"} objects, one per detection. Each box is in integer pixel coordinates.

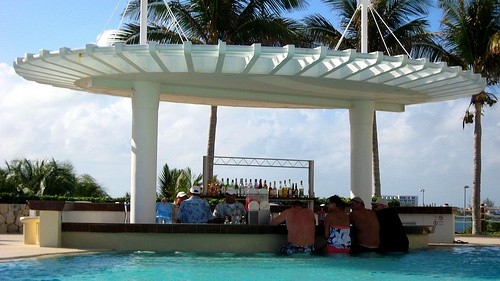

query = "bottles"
[
  {"left": 248, "top": 179, "right": 252, "bottom": 189},
  {"left": 295, "top": 183, "right": 298, "bottom": 198},
  {"left": 292, "top": 183, "right": 295, "bottom": 198},
  {"left": 235, "top": 178, "right": 238, "bottom": 196},
  {"left": 230, "top": 180, "right": 234, "bottom": 196},
  {"left": 225, "top": 178, "right": 230, "bottom": 190},
  {"left": 239, "top": 178, "right": 244, "bottom": 196},
  {"left": 263, "top": 180, "right": 267, "bottom": 189},
  {"left": 287, "top": 179, "right": 292, "bottom": 198},
  {"left": 244, "top": 179, "right": 248, "bottom": 196},
  {"left": 299, "top": 181, "right": 304, "bottom": 196},
  {"left": 278, "top": 181, "right": 283, "bottom": 198},
  {"left": 283, "top": 180, "right": 288, "bottom": 198},
  {"left": 254, "top": 179, "right": 258, "bottom": 194},
  {"left": 258, "top": 179, "right": 262, "bottom": 189},
  {"left": 269, "top": 182, "right": 273, "bottom": 198},
  {"left": 220, "top": 178, "right": 225, "bottom": 193},
  {"left": 273, "top": 181, "right": 277, "bottom": 198}
]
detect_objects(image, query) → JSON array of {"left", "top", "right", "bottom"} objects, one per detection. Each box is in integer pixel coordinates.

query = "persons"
[
  {"left": 160, "top": 191, "right": 189, "bottom": 222},
  {"left": 371, "top": 199, "right": 409, "bottom": 255},
  {"left": 324, "top": 195, "right": 351, "bottom": 255},
  {"left": 346, "top": 197, "right": 379, "bottom": 255},
  {"left": 269, "top": 200, "right": 317, "bottom": 257},
  {"left": 176, "top": 186, "right": 213, "bottom": 223},
  {"left": 212, "top": 187, "right": 246, "bottom": 221}
]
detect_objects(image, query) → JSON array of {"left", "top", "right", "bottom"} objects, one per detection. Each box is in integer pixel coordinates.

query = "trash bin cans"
[{"left": 19, "top": 216, "right": 40, "bottom": 245}]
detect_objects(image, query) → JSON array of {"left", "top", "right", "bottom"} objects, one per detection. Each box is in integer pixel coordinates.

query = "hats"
[
  {"left": 349, "top": 197, "right": 363, "bottom": 204},
  {"left": 189, "top": 186, "right": 201, "bottom": 194},
  {"left": 225, "top": 188, "right": 237, "bottom": 195},
  {"left": 371, "top": 198, "right": 388, "bottom": 207},
  {"left": 177, "top": 191, "right": 189, "bottom": 198}
]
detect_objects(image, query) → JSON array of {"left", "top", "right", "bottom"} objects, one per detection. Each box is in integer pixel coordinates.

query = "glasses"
[{"left": 373, "top": 205, "right": 381, "bottom": 208}]
